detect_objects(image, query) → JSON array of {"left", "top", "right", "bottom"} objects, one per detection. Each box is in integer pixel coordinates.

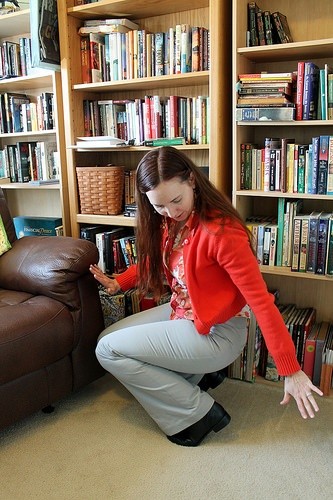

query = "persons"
[{"left": 89, "top": 145, "right": 323, "bottom": 447}]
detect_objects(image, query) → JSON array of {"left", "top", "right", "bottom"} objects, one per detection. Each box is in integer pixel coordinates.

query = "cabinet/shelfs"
[
  {"left": 232, "top": 0, "right": 333, "bottom": 401},
  {"left": 56, "top": 1, "right": 223, "bottom": 239},
  {"left": 0, "top": 7, "right": 70, "bottom": 237}
]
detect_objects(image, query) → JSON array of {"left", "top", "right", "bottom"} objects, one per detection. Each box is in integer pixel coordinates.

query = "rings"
[{"left": 306, "top": 392, "right": 313, "bottom": 398}]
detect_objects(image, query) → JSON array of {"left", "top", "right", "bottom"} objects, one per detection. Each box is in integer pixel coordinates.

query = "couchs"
[{"left": 0, "top": 187, "right": 105, "bottom": 430}]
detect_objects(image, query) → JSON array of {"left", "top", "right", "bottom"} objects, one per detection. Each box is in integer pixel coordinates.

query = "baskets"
[{"left": 76, "top": 165, "right": 125, "bottom": 215}]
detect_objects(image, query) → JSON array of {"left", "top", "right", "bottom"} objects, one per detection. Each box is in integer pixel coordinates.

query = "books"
[
  {"left": 78, "top": 19, "right": 210, "bottom": 84},
  {"left": 0, "top": 38, "right": 32, "bottom": 81},
  {"left": 0, "top": 92, "right": 55, "bottom": 134},
  {"left": 240, "top": 135, "right": 333, "bottom": 195},
  {"left": 123, "top": 167, "right": 135, "bottom": 205},
  {"left": 0, "top": 141, "right": 60, "bottom": 185},
  {"left": 226, "top": 287, "right": 333, "bottom": 397},
  {"left": 245, "top": 197, "right": 333, "bottom": 276},
  {"left": 236, "top": 62, "right": 333, "bottom": 122},
  {"left": 65, "top": 95, "right": 211, "bottom": 149},
  {"left": 246, "top": 2, "right": 293, "bottom": 47},
  {"left": 13, "top": 216, "right": 63, "bottom": 240},
  {"left": 80, "top": 224, "right": 172, "bottom": 314},
  {"left": 74, "top": 0, "right": 101, "bottom": 6}
]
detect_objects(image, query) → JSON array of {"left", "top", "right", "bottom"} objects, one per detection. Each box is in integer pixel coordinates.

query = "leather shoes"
[
  {"left": 166, "top": 400, "right": 231, "bottom": 447},
  {"left": 197, "top": 369, "right": 227, "bottom": 393}
]
